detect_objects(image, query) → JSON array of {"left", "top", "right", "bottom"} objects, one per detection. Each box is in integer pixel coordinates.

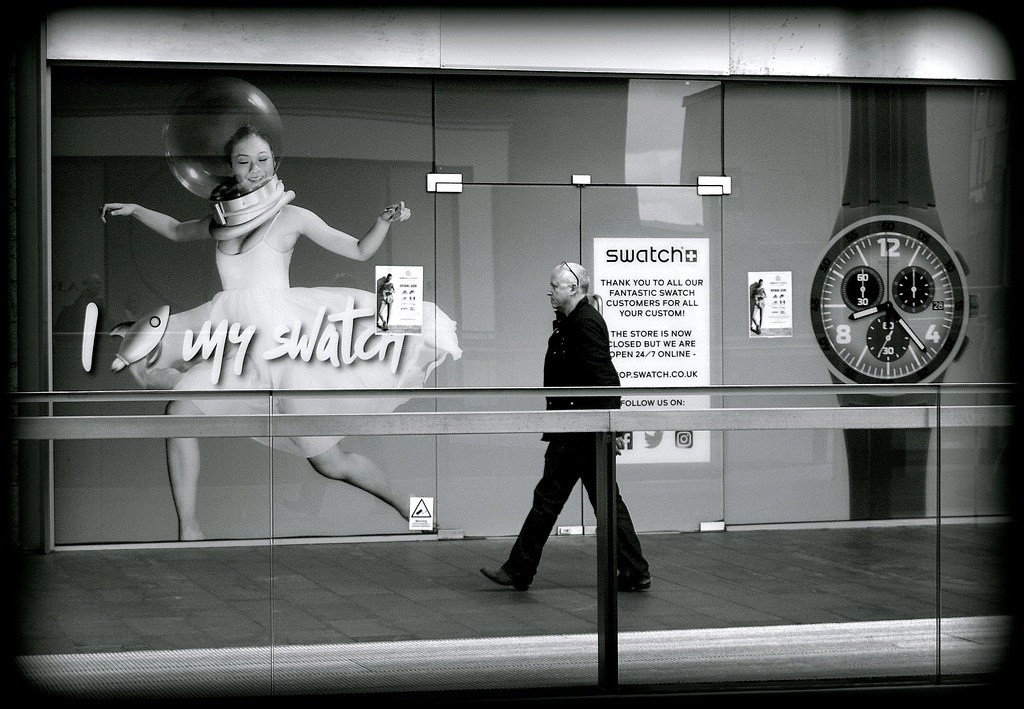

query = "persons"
[
  {"left": 101, "top": 123, "right": 462, "bottom": 540},
  {"left": 480, "top": 261, "right": 652, "bottom": 592},
  {"left": 378, "top": 274, "right": 395, "bottom": 331},
  {"left": 751, "top": 279, "right": 766, "bottom": 335}
]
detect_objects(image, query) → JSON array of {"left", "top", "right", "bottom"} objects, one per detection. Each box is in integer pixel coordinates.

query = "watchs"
[{"left": 810, "top": 83, "right": 978, "bottom": 520}]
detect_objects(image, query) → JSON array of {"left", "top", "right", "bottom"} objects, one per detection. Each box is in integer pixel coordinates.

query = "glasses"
[{"left": 560, "top": 260, "right": 579, "bottom": 285}]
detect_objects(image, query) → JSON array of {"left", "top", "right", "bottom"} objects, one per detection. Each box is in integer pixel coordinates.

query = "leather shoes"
[
  {"left": 481, "top": 564, "right": 532, "bottom": 594},
  {"left": 617, "top": 574, "right": 651, "bottom": 591}
]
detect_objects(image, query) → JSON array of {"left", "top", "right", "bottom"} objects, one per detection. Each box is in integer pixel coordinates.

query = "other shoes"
[{"left": 756, "top": 325, "right": 760, "bottom": 334}]
